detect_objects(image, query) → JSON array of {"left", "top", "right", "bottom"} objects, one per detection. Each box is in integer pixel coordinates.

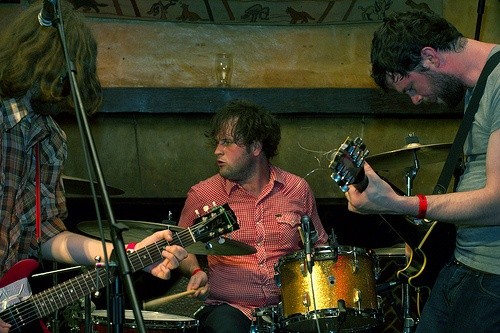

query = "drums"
[
  {"left": 252, "top": 246, "right": 384, "bottom": 333},
  {"left": 76, "top": 310, "right": 194, "bottom": 332}
]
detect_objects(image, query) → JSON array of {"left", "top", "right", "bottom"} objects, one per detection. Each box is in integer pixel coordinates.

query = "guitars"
[
  {"left": 327, "top": 136, "right": 456, "bottom": 319},
  {"left": 0, "top": 199, "right": 240, "bottom": 333}
]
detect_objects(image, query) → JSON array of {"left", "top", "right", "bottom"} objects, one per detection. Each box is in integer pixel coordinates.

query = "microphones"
[
  {"left": 300, "top": 215, "right": 319, "bottom": 244},
  {"left": 38, "top": 0, "right": 58, "bottom": 26}
]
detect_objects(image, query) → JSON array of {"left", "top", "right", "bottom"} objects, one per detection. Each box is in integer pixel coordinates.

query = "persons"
[
  {"left": 177, "top": 104, "right": 329, "bottom": 333},
  {"left": 0, "top": 0, "right": 187, "bottom": 333},
  {"left": 345, "top": 7, "right": 500, "bottom": 333}
]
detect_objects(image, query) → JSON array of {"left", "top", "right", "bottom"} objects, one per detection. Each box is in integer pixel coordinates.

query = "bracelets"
[
  {"left": 191, "top": 269, "right": 202, "bottom": 276},
  {"left": 414, "top": 194, "right": 427, "bottom": 219},
  {"left": 125, "top": 242, "right": 137, "bottom": 254}
]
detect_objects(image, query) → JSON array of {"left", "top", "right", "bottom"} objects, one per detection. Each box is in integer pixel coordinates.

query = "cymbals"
[
  {"left": 74, "top": 218, "right": 256, "bottom": 256},
  {"left": 59, "top": 177, "right": 126, "bottom": 197},
  {"left": 364, "top": 144, "right": 454, "bottom": 174}
]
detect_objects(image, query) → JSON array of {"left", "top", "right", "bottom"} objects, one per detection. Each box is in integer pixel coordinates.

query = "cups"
[{"left": 215, "top": 49, "right": 233, "bottom": 88}]
